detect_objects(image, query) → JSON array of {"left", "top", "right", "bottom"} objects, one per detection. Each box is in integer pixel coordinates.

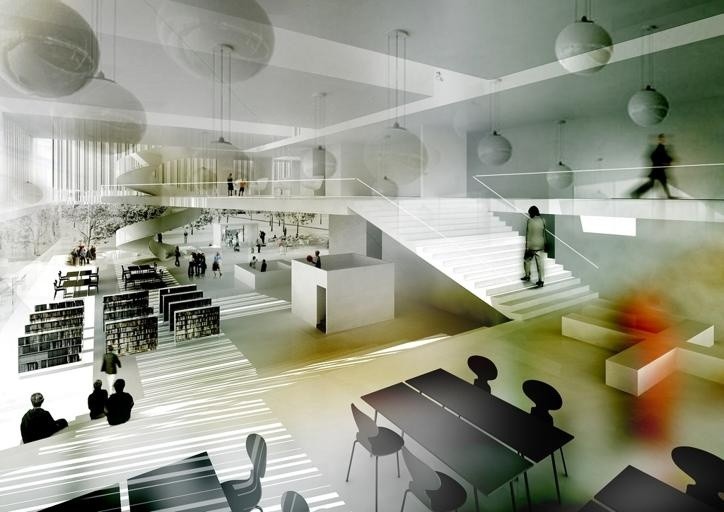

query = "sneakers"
[
  {"left": 520, "top": 276, "right": 531, "bottom": 281},
  {"left": 536, "top": 281, "right": 544, "bottom": 287}
]
